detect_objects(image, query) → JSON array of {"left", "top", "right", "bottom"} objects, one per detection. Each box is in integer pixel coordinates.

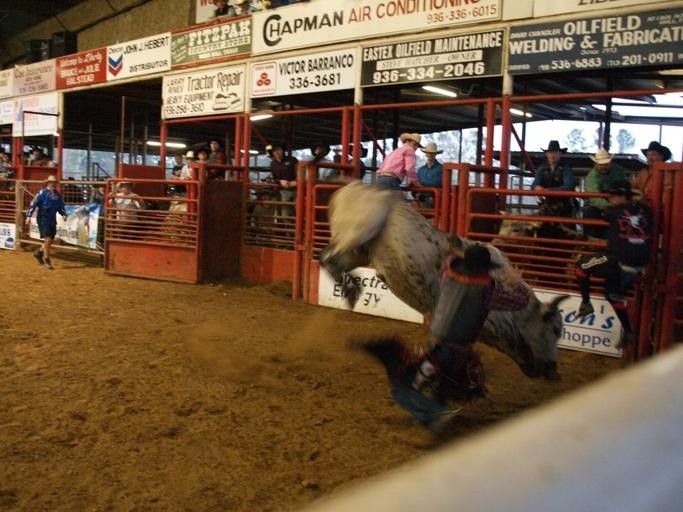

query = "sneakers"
[
  {"left": 615, "top": 331, "right": 636, "bottom": 350},
  {"left": 577, "top": 302, "right": 594, "bottom": 318}
]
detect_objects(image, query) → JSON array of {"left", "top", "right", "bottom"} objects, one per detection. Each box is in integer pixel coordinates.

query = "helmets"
[{"left": 166, "top": 184, "right": 187, "bottom": 196}]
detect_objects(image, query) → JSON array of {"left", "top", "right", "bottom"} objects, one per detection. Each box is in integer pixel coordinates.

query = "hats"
[
  {"left": 451, "top": 243, "right": 503, "bottom": 275},
  {"left": 399, "top": 132, "right": 427, "bottom": 149},
  {"left": 182, "top": 151, "right": 196, "bottom": 161},
  {"left": 600, "top": 179, "right": 641, "bottom": 197},
  {"left": 4, "top": 146, "right": 44, "bottom": 158},
  {"left": 347, "top": 142, "right": 368, "bottom": 158},
  {"left": 421, "top": 142, "right": 443, "bottom": 154},
  {"left": 42, "top": 175, "right": 58, "bottom": 185},
  {"left": 540, "top": 140, "right": 568, "bottom": 153},
  {"left": 115, "top": 177, "right": 136, "bottom": 191},
  {"left": 587, "top": 149, "right": 617, "bottom": 165},
  {"left": 641, "top": 141, "right": 672, "bottom": 162}
]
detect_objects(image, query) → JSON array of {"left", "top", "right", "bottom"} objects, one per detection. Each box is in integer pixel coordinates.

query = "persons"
[
  {"left": 375, "top": 132, "right": 423, "bottom": 199},
  {"left": 412, "top": 143, "right": 444, "bottom": 220},
  {"left": 345, "top": 244, "right": 529, "bottom": 444},
  {"left": 62, "top": 177, "right": 145, "bottom": 241},
  {"left": 629, "top": 140, "right": 673, "bottom": 231},
  {"left": 167, "top": 133, "right": 364, "bottom": 245},
  {"left": 0, "top": 145, "right": 56, "bottom": 187},
  {"left": 583, "top": 148, "right": 626, "bottom": 240},
  {"left": 530, "top": 139, "right": 579, "bottom": 263},
  {"left": 574, "top": 178, "right": 653, "bottom": 349},
  {"left": 25, "top": 175, "right": 68, "bottom": 269}
]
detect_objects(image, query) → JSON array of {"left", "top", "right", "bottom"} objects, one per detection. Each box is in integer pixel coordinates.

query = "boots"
[{"left": 33, "top": 249, "right": 54, "bottom": 269}]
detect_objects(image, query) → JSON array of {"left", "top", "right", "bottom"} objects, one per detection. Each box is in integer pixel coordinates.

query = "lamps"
[
  {"left": 249, "top": 109, "right": 274, "bottom": 122},
  {"left": 422, "top": 82, "right": 459, "bottom": 98},
  {"left": 146, "top": 137, "right": 189, "bottom": 149}
]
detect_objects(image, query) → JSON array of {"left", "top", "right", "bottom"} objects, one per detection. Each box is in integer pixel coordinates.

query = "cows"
[
  {"left": 487, "top": 210, "right": 545, "bottom": 278},
  {"left": 318, "top": 179, "right": 570, "bottom": 383}
]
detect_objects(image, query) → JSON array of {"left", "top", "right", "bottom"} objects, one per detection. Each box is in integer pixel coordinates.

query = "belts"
[{"left": 376, "top": 172, "right": 398, "bottom": 182}]
